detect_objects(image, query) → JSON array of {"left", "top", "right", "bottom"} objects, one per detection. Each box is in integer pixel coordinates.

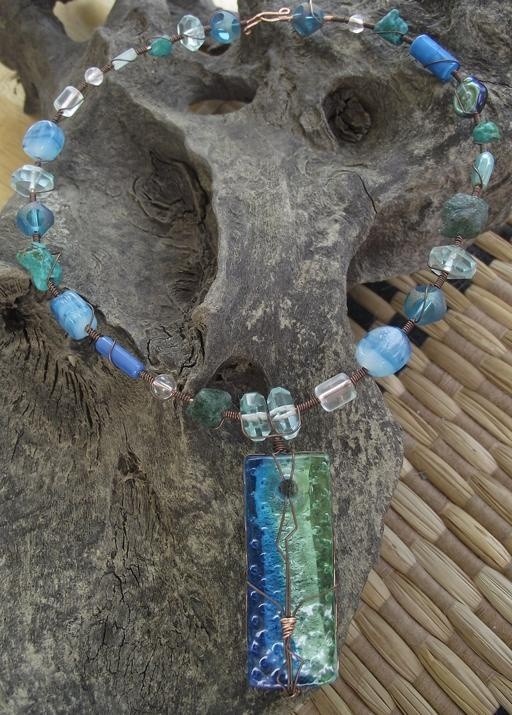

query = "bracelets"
[{"left": 11, "top": 3, "right": 500, "bottom": 696}]
